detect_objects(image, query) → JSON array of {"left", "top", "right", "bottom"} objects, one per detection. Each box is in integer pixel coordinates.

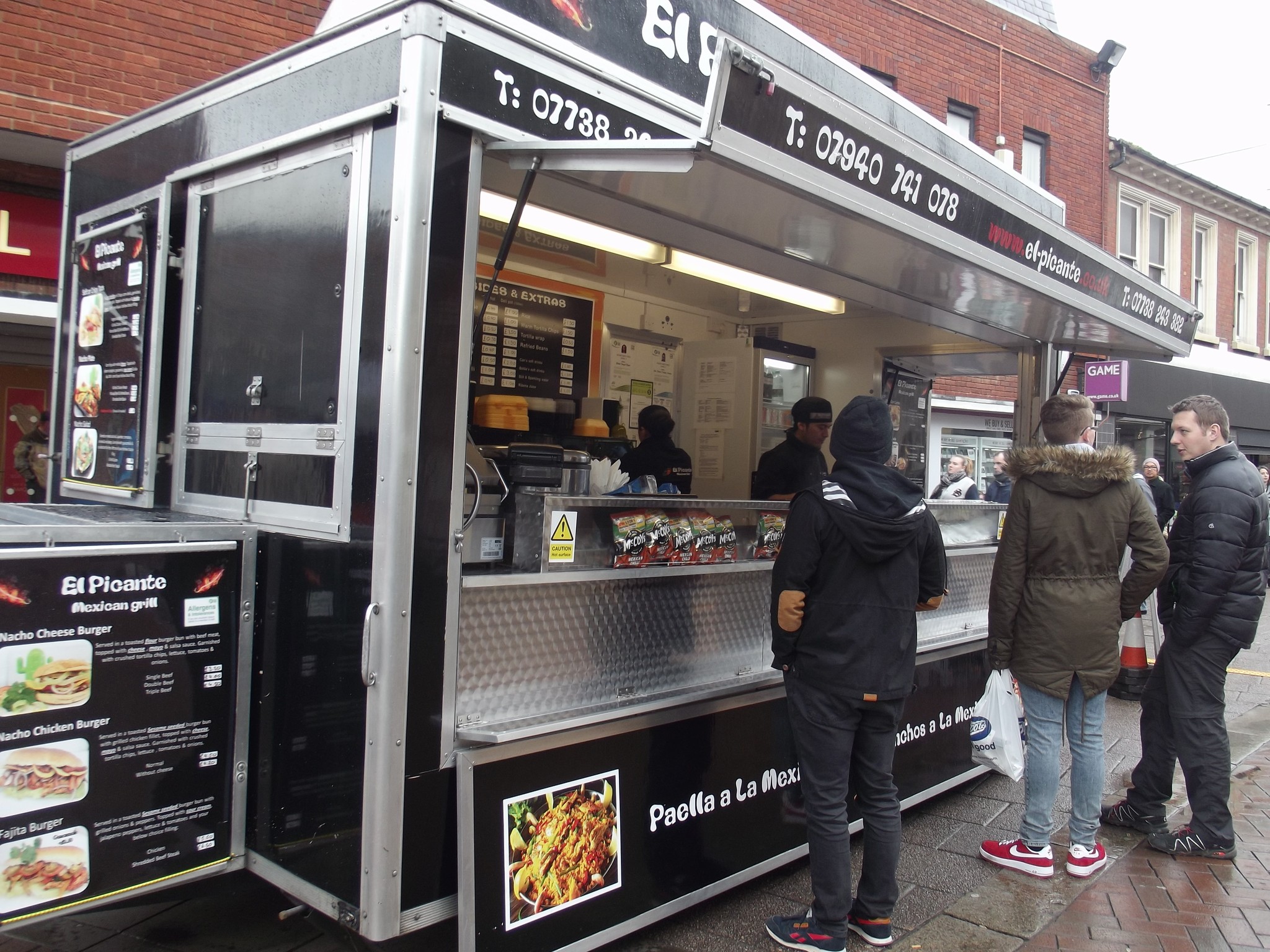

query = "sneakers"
[
  {"left": 847, "top": 896, "right": 893, "bottom": 946},
  {"left": 765, "top": 905, "right": 849, "bottom": 952},
  {"left": 1100, "top": 799, "right": 1169, "bottom": 834},
  {"left": 1066, "top": 840, "right": 1107, "bottom": 877},
  {"left": 979, "top": 839, "right": 1055, "bottom": 879},
  {"left": 1146, "top": 824, "right": 1237, "bottom": 859}
]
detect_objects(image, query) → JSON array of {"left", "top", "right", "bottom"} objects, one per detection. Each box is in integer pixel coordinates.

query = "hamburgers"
[
  {"left": 0, "top": 747, "right": 88, "bottom": 798},
  {"left": 25, "top": 657, "right": 93, "bottom": 706},
  {"left": 0, "top": 844, "right": 91, "bottom": 897}
]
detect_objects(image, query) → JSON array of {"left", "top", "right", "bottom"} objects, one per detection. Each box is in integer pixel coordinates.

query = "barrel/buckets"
[{"left": 476, "top": 443, "right": 590, "bottom": 494}]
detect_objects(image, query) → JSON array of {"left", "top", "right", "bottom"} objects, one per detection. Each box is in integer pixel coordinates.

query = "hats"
[
  {"left": 828, "top": 395, "right": 893, "bottom": 464},
  {"left": 1142, "top": 458, "right": 1160, "bottom": 473},
  {"left": 791, "top": 396, "right": 832, "bottom": 424}
]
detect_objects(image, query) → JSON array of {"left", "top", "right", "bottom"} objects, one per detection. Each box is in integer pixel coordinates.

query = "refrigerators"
[{"left": 680, "top": 335, "right": 816, "bottom": 500}]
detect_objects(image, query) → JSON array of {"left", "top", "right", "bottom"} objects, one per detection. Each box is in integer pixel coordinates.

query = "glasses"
[{"left": 1143, "top": 466, "right": 1157, "bottom": 470}]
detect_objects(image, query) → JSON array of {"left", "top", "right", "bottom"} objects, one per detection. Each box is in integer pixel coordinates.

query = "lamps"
[
  {"left": 1090, "top": 40, "right": 1127, "bottom": 83},
  {"left": 658, "top": 247, "right": 846, "bottom": 316},
  {"left": 479, "top": 188, "right": 669, "bottom": 264}
]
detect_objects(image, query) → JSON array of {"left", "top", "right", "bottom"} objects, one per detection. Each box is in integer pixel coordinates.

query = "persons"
[
  {"left": 977, "top": 392, "right": 1170, "bottom": 879},
  {"left": 769, "top": 394, "right": 950, "bottom": 952},
  {"left": 603, "top": 405, "right": 693, "bottom": 495},
  {"left": 929, "top": 455, "right": 978, "bottom": 500},
  {"left": 1126, "top": 469, "right": 1158, "bottom": 616},
  {"left": 754, "top": 397, "right": 833, "bottom": 501},
  {"left": 13, "top": 410, "right": 50, "bottom": 504},
  {"left": 1094, "top": 394, "right": 1269, "bottom": 859},
  {"left": 1256, "top": 465, "right": 1270, "bottom": 587},
  {"left": 1140, "top": 458, "right": 1175, "bottom": 534},
  {"left": 983, "top": 451, "right": 1011, "bottom": 503}
]
